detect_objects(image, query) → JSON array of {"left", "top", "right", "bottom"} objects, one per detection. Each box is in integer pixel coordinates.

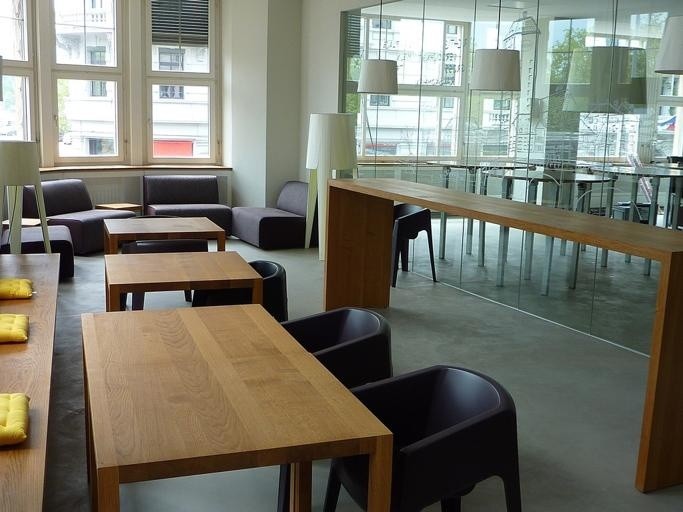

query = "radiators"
[
  {"left": 82, "top": 175, "right": 228, "bottom": 217},
  {"left": 358, "top": 166, "right": 477, "bottom": 214}
]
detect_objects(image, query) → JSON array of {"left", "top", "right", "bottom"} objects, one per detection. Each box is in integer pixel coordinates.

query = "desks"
[
  {"left": 103, "top": 217, "right": 225, "bottom": 254},
  {"left": 425, "top": 161, "right": 683, "bottom": 298},
  {"left": 0, "top": 254, "right": 61, "bottom": 512},
  {"left": 321, "top": 178, "right": 682, "bottom": 493},
  {"left": 80, "top": 304, "right": 395, "bottom": 512},
  {"left": 104, "top": 251, "right": 263, "bottom": 312}
]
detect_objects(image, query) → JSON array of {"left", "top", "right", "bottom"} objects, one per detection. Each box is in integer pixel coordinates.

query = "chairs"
[
  {"left": 120, "top": 215, "right": 208, "bottom": 310},
  {"left": 192, "top": 260, "right": 289, "bottom": 322},
  {"left": 323, "top": 365, "right": 535, "bottom": 512},
  {"left": 280, "top": 306, "right": 393, "bottom": 389}
]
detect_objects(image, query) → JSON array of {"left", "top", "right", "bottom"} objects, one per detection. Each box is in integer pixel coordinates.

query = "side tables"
[
  {"left": 1, "top": 217, "right": 49, "bottom": 227},
  {"left": 95, "top": 202, "right": 143, "bottom": 217}
]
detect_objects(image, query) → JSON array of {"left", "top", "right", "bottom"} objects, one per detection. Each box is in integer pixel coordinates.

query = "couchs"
[
  {"left": 22, "top": 178, "right": 136, "bottom": 256},
  {"left": 232, "top": 181, "right": 309, "bottom": 250},
  {"left": 143, "top": 175, "right": 233, "bottom": 240}
]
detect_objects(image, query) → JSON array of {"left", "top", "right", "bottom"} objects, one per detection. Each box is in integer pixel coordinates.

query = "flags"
[
  {"left": 666, "top": 121, "right": 675, "bottom": 131},
  {"left": 659, "top": 115, "right": 675, "bottom": 127}
]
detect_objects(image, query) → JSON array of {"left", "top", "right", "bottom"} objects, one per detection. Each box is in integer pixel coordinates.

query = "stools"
[
  {"left": 390, "top": 203, "right": 437, "bottom": 288},
  {"left": 0, "top": 225, "right": 74, "bottom": 278}
]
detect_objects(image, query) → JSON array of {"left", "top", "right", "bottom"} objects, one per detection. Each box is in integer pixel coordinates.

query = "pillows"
[
  {"left": 1, "top": 277, "right": 34, "bottom": 299},
  {"left": 0, "top": 392, "right": 31, "bottom": 446},
  {"left": 0, "top": 313, "right": 29, "bottom": 342}
]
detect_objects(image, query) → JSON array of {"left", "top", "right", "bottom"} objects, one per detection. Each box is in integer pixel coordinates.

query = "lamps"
[
  {"left": 469, "top": 1, "right": 522, "bottom": 92},
  {"left": 562, "top": 1, "right": 647, "bottom": 115},
  {"left": 654, "top": 16, "right": 683, "bottom": 75},
  {"left": 357, "top": 1, "right": 398, "bottom": 95}
]
